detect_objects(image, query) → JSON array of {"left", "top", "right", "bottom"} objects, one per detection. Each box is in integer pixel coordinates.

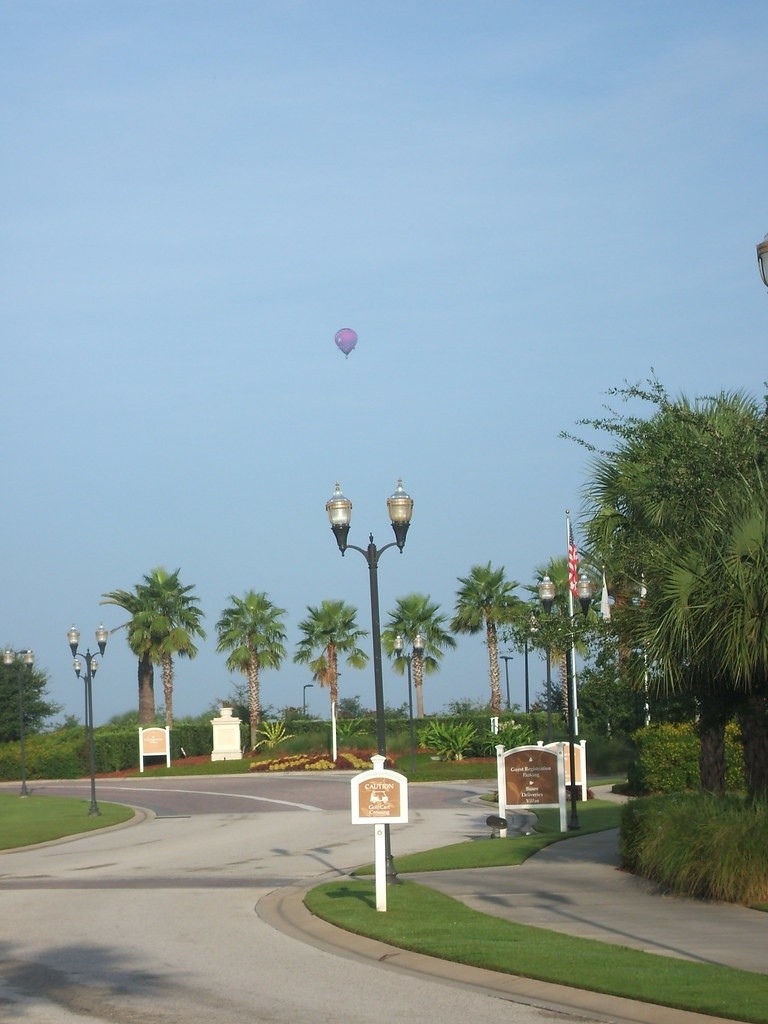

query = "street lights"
[
  {"left": 4, "top": 648, "right": 34, "bottom": 794},
  {"left": 72, "top": 656, "right": 98, "bottom": 772},
  {"left": 540, "top": 571, "right": 591, "bottom": 828},
  {"left": 65, "top": 621, "right": 111, "bottom": 816},
  {"left": 501, "top": 655, "right": 514, "bottom": 708},
  {"left": 325, "top": 480, "right": 414, "bottom": 879},
  {"left": 394, "top": 631, "right": 421, "bottom": 770},
  {"left": 303, "top": 684, "right": 314, "bottom": 715}
]
